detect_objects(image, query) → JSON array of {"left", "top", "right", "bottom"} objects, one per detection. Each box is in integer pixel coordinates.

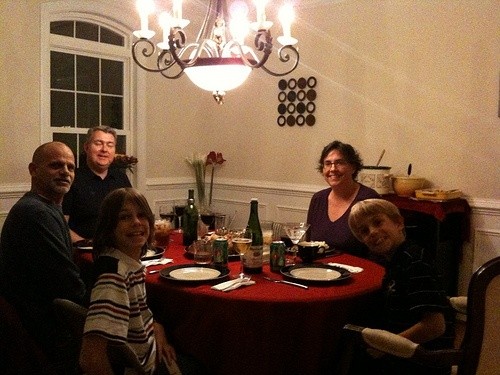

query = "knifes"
[{"left": 263, "top": 276, "right": 308, "bottom": 289}]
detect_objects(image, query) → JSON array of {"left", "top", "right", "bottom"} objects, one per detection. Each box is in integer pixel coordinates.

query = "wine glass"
[
  {"left": 171, "top": 199, "right": 186, "bottom": 233},
  {"left": 201, "top": 206, "right": 214, "bottom": 234},
  {"left": 283, "top": 222, "right": 311, "bottom": 262},
  {"left": 231, "top": 227, "right": 252, "bottom": 280}
]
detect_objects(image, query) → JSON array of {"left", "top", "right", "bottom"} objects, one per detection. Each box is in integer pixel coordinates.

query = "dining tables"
[{"left": 77, "top": 229, "right": 385, "bottom": 375}]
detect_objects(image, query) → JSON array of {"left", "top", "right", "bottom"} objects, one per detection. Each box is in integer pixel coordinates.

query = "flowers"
[{"left": 187, "top": 150, "right": 227, "bottom": 207}]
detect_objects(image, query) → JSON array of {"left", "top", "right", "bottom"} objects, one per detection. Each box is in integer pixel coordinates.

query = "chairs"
[
  {"left": 345, "top": 256, "right": 500, "bottom": 375},
  {"left": 0, "top": 294, "right": 146, "bottom": 375}
]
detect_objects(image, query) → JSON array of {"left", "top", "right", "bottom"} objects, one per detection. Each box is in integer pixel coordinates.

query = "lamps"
[{"left": 132, "top": 0, "right": 300, "bottom": 104}]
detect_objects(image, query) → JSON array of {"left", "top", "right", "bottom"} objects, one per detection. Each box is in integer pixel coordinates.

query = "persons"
[
  {"left": 0, "top": 125, "right": 75, "bottom": 365},
  {"left": 349, "top": 199, "right": 457, "bottom": 375},
  {"left": 62, "top": 125, "right": 134, "bottom": 242},
  {"left": 80, "top": 188, "right": 176, "bottom": 375},
  {"left": 305, "top": 140, "right": 379, "bottom": 260}
]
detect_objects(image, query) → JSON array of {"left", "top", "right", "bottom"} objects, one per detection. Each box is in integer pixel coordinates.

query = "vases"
[{"left": 199, "top": 205, "right": 216, "bottom": 215}]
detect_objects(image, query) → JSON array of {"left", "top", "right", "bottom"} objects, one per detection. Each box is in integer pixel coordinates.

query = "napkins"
[
  {"left": 141, "top": 257, "right": 175, "bottom": 266},
  {"left": 328, "top": 262, "right": 364, "bottom": 274},
  {"left": 211, "top": 273, "right": 255, "bottom": 292}
]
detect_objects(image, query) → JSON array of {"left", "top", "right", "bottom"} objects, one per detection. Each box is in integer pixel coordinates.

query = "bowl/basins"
[{"left": 390, "top": 176, "right": 427, "bottom": 197}]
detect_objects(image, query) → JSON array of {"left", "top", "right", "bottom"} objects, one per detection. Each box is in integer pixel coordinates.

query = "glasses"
[{"left": 322, "top": 159, "right": 351, "bottom": 169}]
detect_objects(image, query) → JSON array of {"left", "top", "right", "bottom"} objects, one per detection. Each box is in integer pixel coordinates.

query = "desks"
[
  {"left": 160, "top": 212, "right": 217, "bottom": 232},
  {"left": 379, "top": 194, "right": 470, "bottom": 296}
]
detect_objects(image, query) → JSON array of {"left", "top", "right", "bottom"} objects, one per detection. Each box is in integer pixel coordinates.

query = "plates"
[
  {"left": 159, "top": 264, "right": 231, "bottom": 284},
  {"left": 76, "top": 243, "right": 93, "bottom": 252},
  {"left": 140, "top": 246, "right": 165, "bottom": 261},
  {"left": 279, "top": 263, "right": 351, "bottom": 284},
  {"left": 185, "top": 242, "right": 269, "bottom": 259}
]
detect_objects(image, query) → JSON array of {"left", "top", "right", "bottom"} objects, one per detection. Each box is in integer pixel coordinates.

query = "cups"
[
  {"left": 151, "top": 220, "right": 169, "bottom": 248},
  {"left": 215, "top": 216, "right": 229, "bottom": 235},
  {"left": 297, "top": 242, "right": 326, "bottom": 263},
  {"left": 159, "top": 204, "right": 174, "bottom": 222},
  {"left": 193, "top": 239, "right": 213, "bottom": 264}
]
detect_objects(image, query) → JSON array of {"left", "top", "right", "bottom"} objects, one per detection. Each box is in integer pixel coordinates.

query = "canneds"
[
  {"left": 212, "top": 238, "right": 229, "bottom": 267},
  {"left": 270, "top": 240, "right": 286, "bottom": 272}
]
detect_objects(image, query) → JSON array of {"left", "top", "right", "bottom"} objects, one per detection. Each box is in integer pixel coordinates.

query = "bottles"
[
  {"left": 242, "top": 198, "right": 263, "bottom": 275},
  {"left": 183, "top": 190, "right": 198, "bottom": 244}
]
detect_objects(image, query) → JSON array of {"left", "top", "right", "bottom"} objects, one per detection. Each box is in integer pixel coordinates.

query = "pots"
[{"left": 357, "top": 165, "right": 391, "bottom": 195}]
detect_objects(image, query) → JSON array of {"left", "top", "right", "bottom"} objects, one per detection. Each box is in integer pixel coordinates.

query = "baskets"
[{"left": 415, "top": 189, "right": 461, "bottom": 200}]
